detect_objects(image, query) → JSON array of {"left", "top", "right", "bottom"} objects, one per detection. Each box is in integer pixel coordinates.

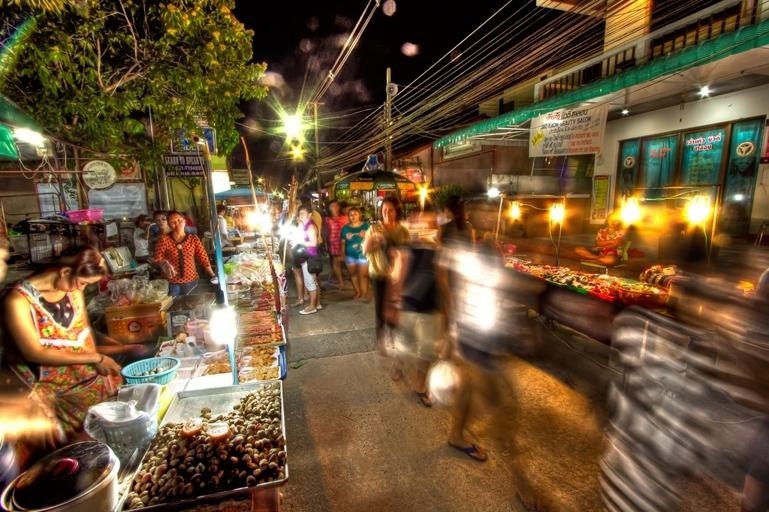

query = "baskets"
[
  {"left": 119, "top": 356, "right": 181, "bottom": 386},
  {"left": 94, "top": 382, "right": 164, "bottom": 468}
]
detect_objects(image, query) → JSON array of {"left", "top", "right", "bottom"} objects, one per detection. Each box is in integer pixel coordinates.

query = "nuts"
[{"left": 123, "top": 382, "right": 286, "bottom": 509}]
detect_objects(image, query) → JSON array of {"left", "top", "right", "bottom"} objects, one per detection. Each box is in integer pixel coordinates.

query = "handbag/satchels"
[
  {"left": 307, "top": 256, "right": 323, "bottom": 273},
  {"left": 291, "top": 244, "right": 308, "bottom": 264}
]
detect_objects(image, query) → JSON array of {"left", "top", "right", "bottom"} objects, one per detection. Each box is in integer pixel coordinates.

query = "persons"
[
  {"left": 181, "top": 210, "right": 195, "bottom": 233},
  {"left": 209, "top": 193, "right": 394, "bottom": 355},
  {"left": 155, "top": 210, "right": 214, "bottom": 296},
  {"left": 92, "top": 326, "right": 148, "bottom": 365},
  {"left": 393, "top": 196, "right": 769, "bottom": 510},
  {"left": 133, "top": 214, "right": 152, "bottom": 263},
  {"left": 1, "top": 245, "right": 56, "bottom": 493},
  {"left": 148, "top": 211, "right": 173, "bottom": 265},
  {"left": 1, "top": 239, "right": 126, "bottom": 442}
]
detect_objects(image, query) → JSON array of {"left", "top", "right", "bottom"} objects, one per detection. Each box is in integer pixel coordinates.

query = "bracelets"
[{"left": 93, "top": 353, "right": 104, "bottom": 365}]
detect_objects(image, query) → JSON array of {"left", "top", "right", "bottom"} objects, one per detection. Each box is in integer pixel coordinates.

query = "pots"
[{"left": 0, "top": 439, "right": 121, "bottom": 511}]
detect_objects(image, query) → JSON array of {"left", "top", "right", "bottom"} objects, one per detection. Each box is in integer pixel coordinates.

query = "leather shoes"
[
  {"left": 298, "top": 306, "right": 317, "bottom": 314},
  {"left": 317, "top": 304, "right": 323, "bottom": 310}
]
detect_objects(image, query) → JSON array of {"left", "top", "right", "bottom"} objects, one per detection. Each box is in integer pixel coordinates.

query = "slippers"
[
  {"left": 447, "top": 437, "right": 488, "bottom": 462},
  {"left": 516, "top": 488, "right": 544, "bottom": 511},
  {"left": 415, "top": 388, "right": 436, "bottom": 407}
]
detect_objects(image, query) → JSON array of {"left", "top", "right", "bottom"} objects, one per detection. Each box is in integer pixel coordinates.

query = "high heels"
[
  {"left": 303, "top": 294, "right": 310, "bottom": 302},
  {"left": 289, "top": 299, "right": 305, "bottom": 308}
]
